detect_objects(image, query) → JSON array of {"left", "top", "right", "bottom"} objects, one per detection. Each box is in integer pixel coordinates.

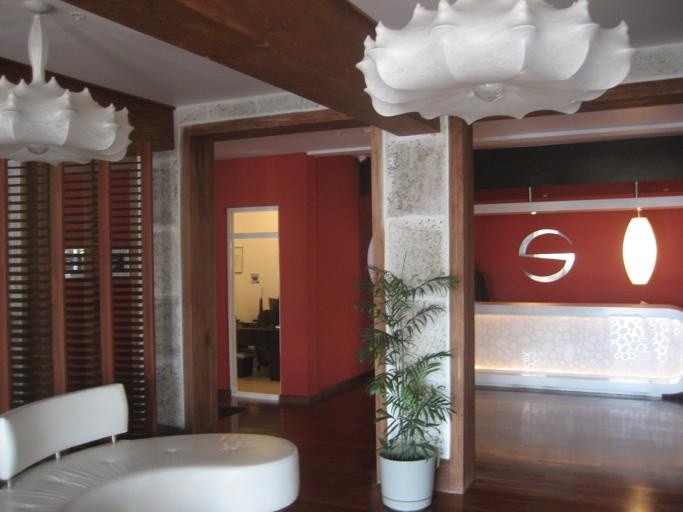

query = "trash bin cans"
[{"left": 236, "top": 352, "right": 252, "bottom": 377}]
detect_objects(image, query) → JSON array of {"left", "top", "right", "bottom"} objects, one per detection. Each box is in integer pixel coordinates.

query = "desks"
[{"left": 236, "top": 322, "right": 278, "bottom": 381}]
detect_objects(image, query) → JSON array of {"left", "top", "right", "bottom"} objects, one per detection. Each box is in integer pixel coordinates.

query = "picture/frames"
[{"left": 233, "top": 246, "right": 243, "bottom": 274}]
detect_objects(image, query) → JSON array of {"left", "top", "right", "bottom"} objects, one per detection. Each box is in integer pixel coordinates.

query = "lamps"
[
  {"left": 0, "top": 0, "right": 137, "bottom": 166},
  {"left": 355, "top": 0, "right": 637, "bottom": 124},
  {"left": 621, "top": 208, "right": 657, "bottom": 286}
]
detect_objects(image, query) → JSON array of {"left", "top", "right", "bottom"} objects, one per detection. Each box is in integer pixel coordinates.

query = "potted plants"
[{"left": 349, "top": 252, "right": 459, "bottom": 510}]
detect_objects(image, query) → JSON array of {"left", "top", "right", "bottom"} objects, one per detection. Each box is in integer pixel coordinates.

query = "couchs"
[{"left": 0, "top": 383, "right": 300, "bottom": 511}]
